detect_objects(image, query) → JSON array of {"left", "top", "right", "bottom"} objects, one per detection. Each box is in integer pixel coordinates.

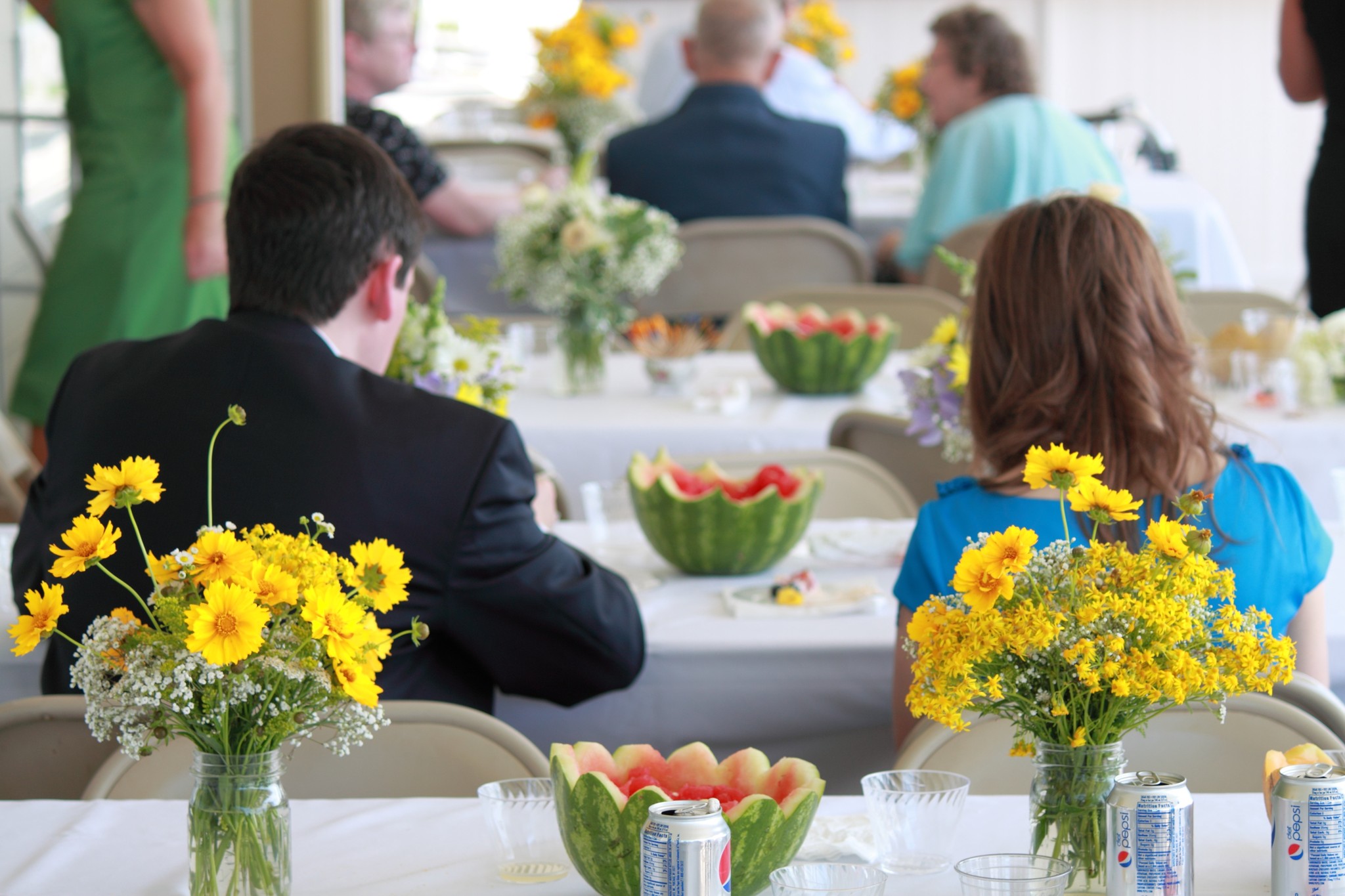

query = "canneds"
[
  {"left": 1269, "top": 763, "right": 1345, "bottom": 896},
  {"left": 1105, "top": 770, "right": 1195, "bottom": 896},
  {"left": 640, "top": 798, "right": 732, "bottom": 896}
]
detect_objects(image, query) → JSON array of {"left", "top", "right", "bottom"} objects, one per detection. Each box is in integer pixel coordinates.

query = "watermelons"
[
  {"left": 745, "top": 300, "right": 898, "bottom": 394},
  {"left": 626, "top": 448, "right": 819, "bottom": 574},
  {"left": 550, "top": 741, "right": 826, "bottom": 896}
]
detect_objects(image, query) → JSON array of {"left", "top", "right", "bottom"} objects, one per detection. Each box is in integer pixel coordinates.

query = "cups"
[
  {"left": 477, "top": 777, "right": 571, "bottom": 883},
  {"left": 769, "top": 863, "right": 887, "bottom": 896},
  {"left": 860, "top": 769, "right": 971, "bottom": 875},
  {"left": 582, "top": 477, "right": 636, "bottom": 548},
  {"left": 954, "top": 853, "right": 1073, "bottom": 896},
  {"left": 510, "top": 320, "right": 558, "bottom": 372}
]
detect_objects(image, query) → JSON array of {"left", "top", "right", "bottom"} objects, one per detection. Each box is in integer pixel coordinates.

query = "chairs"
[{"left": 0, "top": 107, "right": 1345, "bottom": 799}]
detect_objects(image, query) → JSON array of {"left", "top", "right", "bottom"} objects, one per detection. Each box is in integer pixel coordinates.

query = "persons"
[
  {"left": 9, "top": 0, "right": 1345, "bottom": 465},
  {"left": 890, "top": 191, "right": 1332, "bottom": 740},
  {"left": 9, "top": 122, "right": 648, "bottom": 717}
]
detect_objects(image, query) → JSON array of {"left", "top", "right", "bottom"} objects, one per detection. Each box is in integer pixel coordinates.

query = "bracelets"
[{"left": 189, "top": 191, "right": 218, "bottom": 209}]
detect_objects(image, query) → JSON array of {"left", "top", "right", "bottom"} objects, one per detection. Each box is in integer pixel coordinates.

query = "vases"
[
  {"left": 177, "top": 746, "right": 292, "bottom": 896},
  {"left": 564, "top": 317, "right": 611, "bottom": 395},
  {"left": 1029, "top": 741, "right": 1128, "bottom": 896}
]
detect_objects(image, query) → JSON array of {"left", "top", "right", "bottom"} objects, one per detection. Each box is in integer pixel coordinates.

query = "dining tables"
[
  {"left": 493, "top": 349, "right": 1345, "bottom": 519},
  {"left": 0, "top": 495, "right": 1345, "bottom": 762},
  {"left": 0, "top": 792, "right": 1280, "bottom": 896}
]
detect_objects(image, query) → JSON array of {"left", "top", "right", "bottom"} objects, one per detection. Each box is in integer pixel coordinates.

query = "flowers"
[
  {"left": 7, "top": 404, "right": 430, "bottom": 896},
  {"left": 877, "top": 59, "right": 936, "bottom": 163},
  {"left": 523, "top": 13, "right": 640, "bottom": 172},
  {"left": 496, "top": 183, "right": 682, "bottom": 373},
  {"left": 787, "top": 0, "right": 851, "bottom": 64},
  {"left": 904, "top": 444, "right": 1296, "bottom": 896},
  {"left": 387, "top": 277, "right": 508, "bottom": 419},
  {"left": 898, "top": 240, "right": 977, "bottom": 462}
]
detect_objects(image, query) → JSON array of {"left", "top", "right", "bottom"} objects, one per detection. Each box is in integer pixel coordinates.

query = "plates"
[{"left": 721, "top": 578, "right": 874, "bottom": 614}]
